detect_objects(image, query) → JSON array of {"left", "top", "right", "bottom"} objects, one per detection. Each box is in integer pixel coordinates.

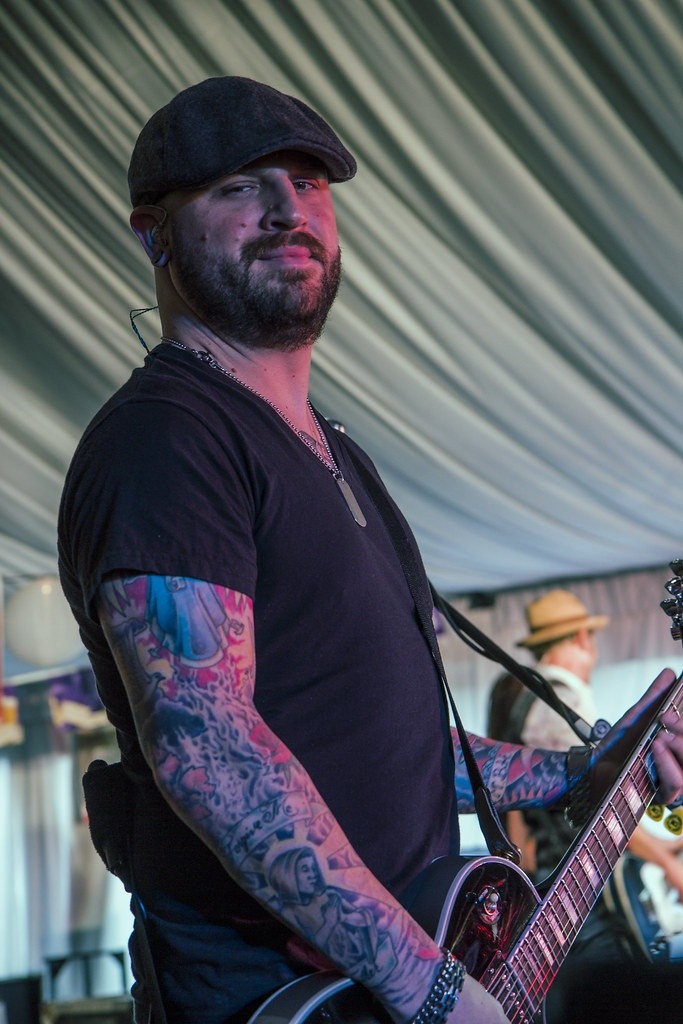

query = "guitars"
[{"left": 246, "top": 559, "right": 683, "bottom": 1023}]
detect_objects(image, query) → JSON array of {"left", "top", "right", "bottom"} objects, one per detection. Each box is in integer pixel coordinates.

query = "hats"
[
  {"left": 126, "top": 76, "right": 357, "bottom": 206},
  {"left": 516, "top": 588, "right": 609, "bottom": 646}
]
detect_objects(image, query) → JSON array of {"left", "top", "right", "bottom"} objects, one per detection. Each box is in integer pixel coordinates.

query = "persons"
[
  {"left": 55, "top": 76, "right": 683, "bottom": 1024},
  {"left": 488, "top": 585, "right": 613, "bottom": 890}
]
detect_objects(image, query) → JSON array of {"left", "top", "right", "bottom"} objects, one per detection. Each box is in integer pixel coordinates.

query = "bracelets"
[
  {"left": 565, "top": 745, "right": 595, "bottom": 792},
  {"left": 407, "top": 946, "right": 467, "bottom": 1024}
]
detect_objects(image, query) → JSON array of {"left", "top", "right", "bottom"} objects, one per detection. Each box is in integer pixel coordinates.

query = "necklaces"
[{"left": 160, "top": 335, "right": 367, "bottom": 528}]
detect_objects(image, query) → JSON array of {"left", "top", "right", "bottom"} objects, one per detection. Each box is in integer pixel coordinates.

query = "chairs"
[{"left": 43, "top": 949, "right": 133, "bottom": 1024}]
